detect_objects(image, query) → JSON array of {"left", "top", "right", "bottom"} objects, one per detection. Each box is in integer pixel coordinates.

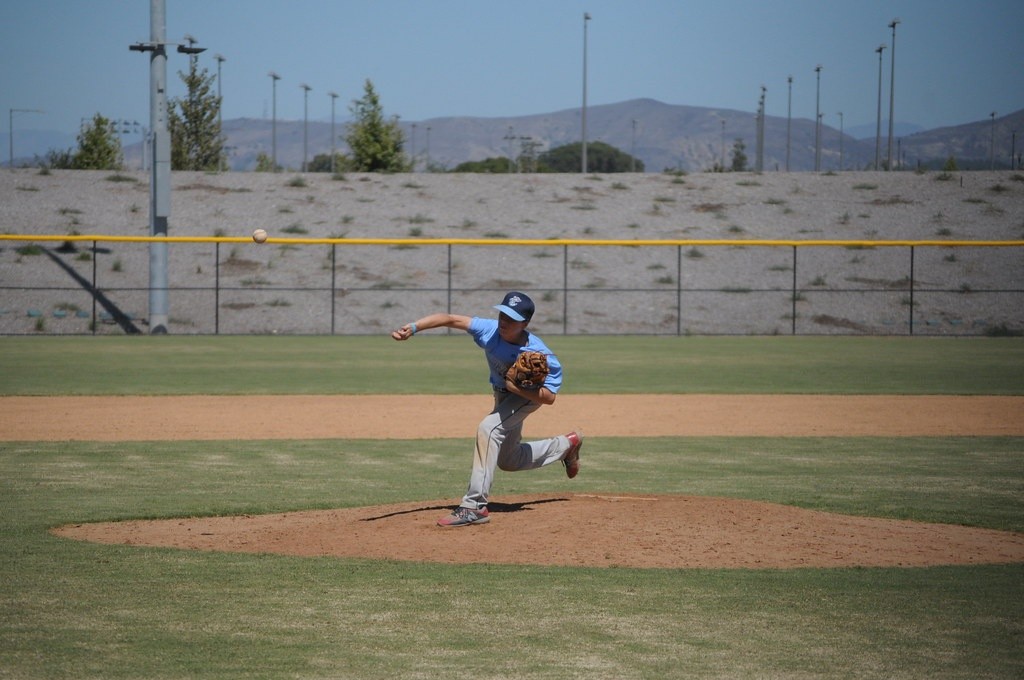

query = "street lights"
[
  {"left": 328, "top": 91, "right": 340, "bottom": 172},
  {"left": 989, "top": 111, "right": 997, "bottom": 171},
  {"left": 887, "top": 16, "right": 901, "bottom": 172},
  {"left": 581, "top": 13, "right": 593, "bottom": 173},
  {"left": 814, "top": 62, "right": 822, "bottom": 171},
  {"left": 267, "top": 72, "right": 281, "bottom": 169},
  {"left": 876, "top": 43, "right": 889, "bottom": 171},
  {"left": 300, "top": 82, "right": 312, "bottom": 173},
  {"left": 786, "top": 74, "right": 794, "bottom": 172},
  {"left": 425, "top": 126, "right": 431, "bottom": 161},
  {"left": 720, "top": 119, "right": 726, "bottom": 172},
  {"left": 213, "top": 52, "right": 226, "bottom": 172},
  {"left": 353, "top": 98, "right": 365, "bottom": 129},
  {"left": 631, "top": 118, "right": 637, "bottom": 172},
  {"left": 837, "top": 113, "right": 844, "bottom": 172},
  {"left": 411, "top": 124, "right": 417, "bottom": 160},
  {"left": 184, "top": 32, "right": 200, "bottom": 168}
]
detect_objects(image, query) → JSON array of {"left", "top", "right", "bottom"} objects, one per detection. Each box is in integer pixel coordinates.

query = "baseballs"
[{"left": 253, "top": 229, "right": 267, "bottom": 243}]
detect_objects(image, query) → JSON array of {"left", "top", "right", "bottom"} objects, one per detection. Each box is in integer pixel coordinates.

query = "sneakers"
[
  {"left": 436, "top": 506, "right": 491, "bottom": 528},
  {"left": 560, "top": 430, "right": 583, "bottom": 479}
]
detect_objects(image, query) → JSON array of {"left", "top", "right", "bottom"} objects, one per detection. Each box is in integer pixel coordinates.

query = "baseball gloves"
[{"left": 505, "top": 350, "right": 558, "bottom": 391}]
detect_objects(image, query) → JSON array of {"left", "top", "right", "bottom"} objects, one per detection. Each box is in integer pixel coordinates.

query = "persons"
[{"left": 392, "top": 291, "right": 583, "bottom": 529}]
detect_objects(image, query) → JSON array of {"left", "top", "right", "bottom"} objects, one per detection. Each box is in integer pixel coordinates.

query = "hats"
[{"left": 492, "top": 291, "right": 536, "bottom": 322}]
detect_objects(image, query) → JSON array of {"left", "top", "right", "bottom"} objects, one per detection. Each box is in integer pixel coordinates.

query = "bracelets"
[{"left": 410, "top": 322, "right": 417, "bottom": 334}]
